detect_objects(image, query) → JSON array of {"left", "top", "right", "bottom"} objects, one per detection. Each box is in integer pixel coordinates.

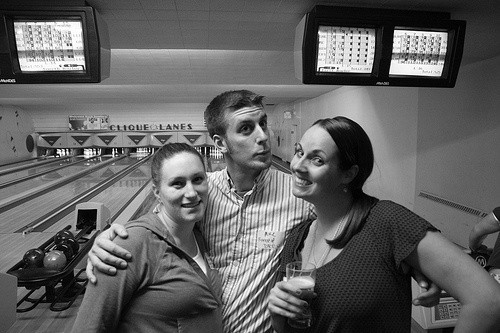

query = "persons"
[
  {"left": 71, "top": 142, "right": 225, "bottom": 333},
  {"left": 85, "top": 91, "right": 442, "bottom": 333},
  {"left": 268, "top": 113, "right": 500, "bottom": 333},
  {"left": 469, "top": 206, "right": 500, "bottom": 272}
]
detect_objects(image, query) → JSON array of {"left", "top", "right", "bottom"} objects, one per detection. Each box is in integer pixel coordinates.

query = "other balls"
[
  {"left": 23, "top": 248, "right": 45, "bottom": 269},
  {"left": 42, "top": 250, "right": 67, "bottom": 271},
  {"left": 53, "top": 230, "right": 80, "bottom": 258}
]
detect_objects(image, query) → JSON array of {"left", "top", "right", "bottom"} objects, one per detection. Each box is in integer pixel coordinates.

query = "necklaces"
[{"left": 299, "top": 211, "right": 347, "bottom": 277}]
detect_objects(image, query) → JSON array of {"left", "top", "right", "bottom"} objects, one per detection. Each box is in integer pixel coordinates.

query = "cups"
[{"left": 286, "top": 262, "right": 316, "bottom": 329}]
